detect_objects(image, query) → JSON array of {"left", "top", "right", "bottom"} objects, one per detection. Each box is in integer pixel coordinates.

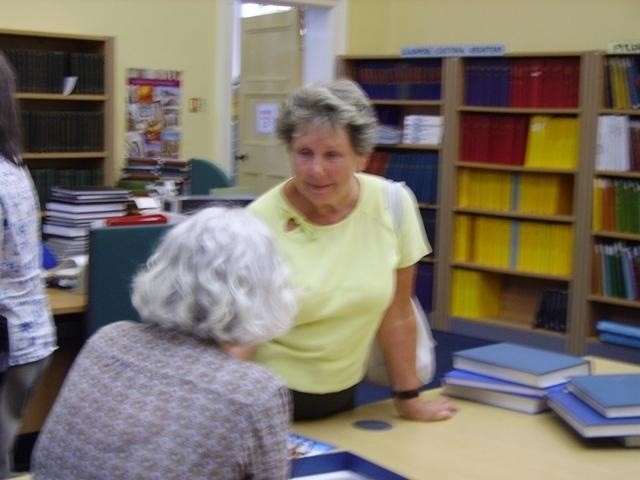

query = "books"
[
  {"left": 592, "top": 53, "right": 640, "bottom": 347},
  {"left": 445, "top": 342, "right": 640, "bottom": 447},
  {"left": 361, "top": 68, "right": 442, "bottom": 312},
  {"left": 43, "top": 156, "right": 191, "bottom": 285},
  {"left": 452, "top": 61, "right": 578, "bottom": 334}
]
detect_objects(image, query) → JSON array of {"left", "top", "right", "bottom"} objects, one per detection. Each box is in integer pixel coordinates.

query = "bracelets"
[{"left": 392, "top": 389, "right": 419, "bottom": 398}]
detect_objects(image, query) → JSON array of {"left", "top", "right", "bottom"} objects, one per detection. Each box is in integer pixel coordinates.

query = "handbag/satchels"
[{"left": 368, "top": 297, "right": 435, "bottom": 389}]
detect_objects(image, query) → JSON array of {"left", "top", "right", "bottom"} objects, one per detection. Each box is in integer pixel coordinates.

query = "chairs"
[{"left": 87, "top": 225, "right": 176, "bottom": 344}]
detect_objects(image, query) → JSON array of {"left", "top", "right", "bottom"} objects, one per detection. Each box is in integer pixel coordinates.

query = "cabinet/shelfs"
[
  {"left": 336, "top": 54, "right": 640, "bottom": 365},
  {"left": 0, "top": 29, "right": 116, "bottom": 219}
]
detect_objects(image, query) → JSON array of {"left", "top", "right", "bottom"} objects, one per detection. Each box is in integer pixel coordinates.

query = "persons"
[
  {"left": 240, "top": 76, "right": 458, "bottom": 422},
  {"left": 0, "top": 55, "right": 57, "bottom": 479},
  {"left": 29, "top": 208, "right": 299, "bottom": 479}
]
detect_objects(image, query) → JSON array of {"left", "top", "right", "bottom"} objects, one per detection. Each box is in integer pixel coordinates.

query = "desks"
[
  {"left": 289, "top": 355, "right": 639, "bottom": 480},
  {"left": 15, "top": 287, "right": 88, "bottom": 472}
]
